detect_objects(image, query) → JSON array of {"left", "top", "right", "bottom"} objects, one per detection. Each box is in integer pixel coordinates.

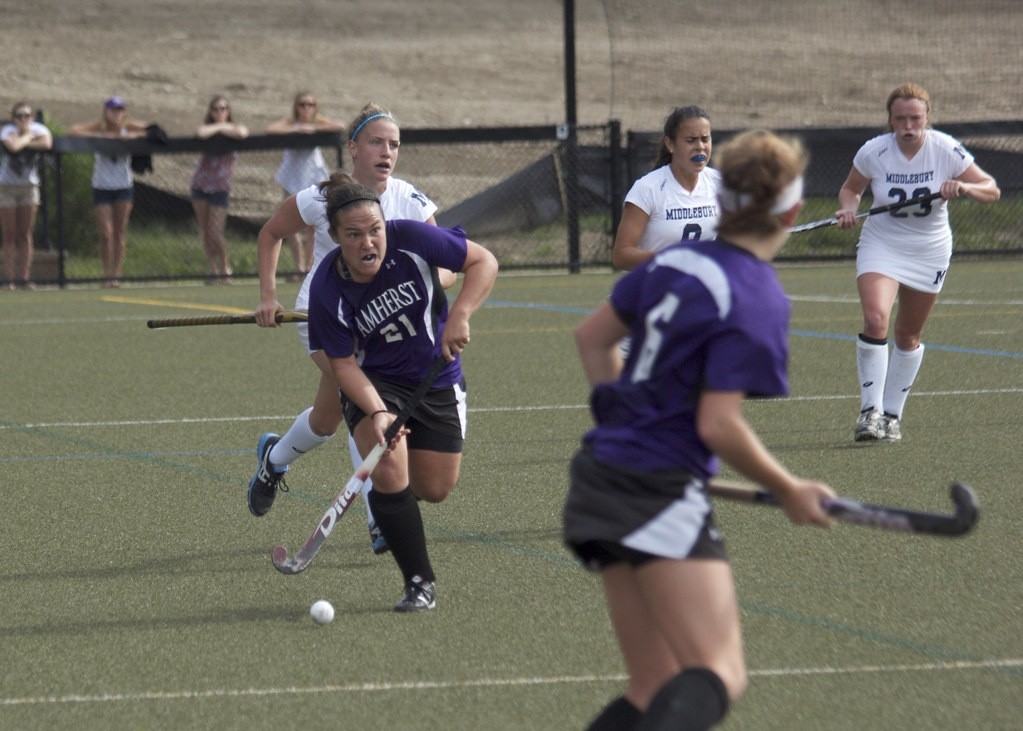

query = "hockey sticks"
[
  {"left": 270, "top": 350, "right": 452, "bottom": 576},
  {"left": 791, "top": 190, "right": 942, "bottom": 235},
  {"left": 144, "top": 310, "right": 309, "bottom": 328},
  {"left": 710, "top": 478, "right": 983, "bottom": 539}
]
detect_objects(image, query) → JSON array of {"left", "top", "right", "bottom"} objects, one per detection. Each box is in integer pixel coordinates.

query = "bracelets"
[{"left": 371, "top": 410, "right": 388, "bottom": 420}]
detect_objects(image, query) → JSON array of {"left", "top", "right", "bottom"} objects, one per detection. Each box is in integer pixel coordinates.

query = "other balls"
[{"left": 310, "top": 599, "right": 335, "bottom": 626}]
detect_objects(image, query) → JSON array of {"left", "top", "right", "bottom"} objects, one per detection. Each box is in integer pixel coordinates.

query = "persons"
[
  {"left": 265, "top": 91, "right": 345, "bottom": 282},
  {"left": 0, "top": 102, "right": 53, "bottom": 291},
  {"left": 67, "top": 97, "right": 148, "bottom": 286},
  {"left": 561, "top": 128, "right": 836, "bottom": 730},
  {"left": 247, "top": 101, "right": 457, "bottom": 555},
  {"left": 189, "top": 94, "right": 251, "bottom": 285},
  {"left": 310, "top": 172, "right": 500, "bottom": 612},
  {"left": 834, "top": 85, "right": 1000, "bottom": 443},
  {"left": 613, "top": 105, "right": 721, "bottom": 270}
]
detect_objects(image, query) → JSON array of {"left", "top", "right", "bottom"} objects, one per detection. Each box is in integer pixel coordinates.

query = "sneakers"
[
  {"left": 247, "top": 433, "right": 289, "bottom": 517},
  {"left": 854, "top": 406, "right": 885, "bottom": 441},
  {"left": 883, "top": 410, "right": 902, "bottom": 440},
  {"left": 368, "top": 524, "right": 390, "bottom": 554},
  {"left": 393, "top": 575, "right": 436, "bottom": 613}
]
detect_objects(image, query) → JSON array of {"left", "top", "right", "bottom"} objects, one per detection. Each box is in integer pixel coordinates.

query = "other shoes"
[
  {"left": 205, "top": 272, "right": 222, "bottom": 285},
  {"left": 292, "top": 270, "right": 309, "bottom": 279},
  {"left": 103, "top": 276, "right": 120, "bottom": 288},
  {"left": 1, "top": 282, "right": 17, "bottom": 292},
  {"left": 224, "top": 269, "right": 233, "bottom": 284},
  {"left": 22, "top": 281, "right": 38, "bottom": 291}
]
